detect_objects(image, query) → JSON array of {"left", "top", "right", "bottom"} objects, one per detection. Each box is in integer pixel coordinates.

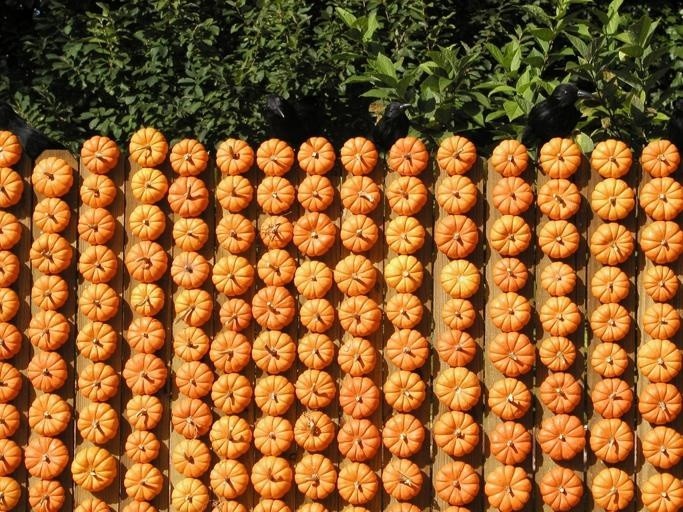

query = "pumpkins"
[{"left": 0, "top": 128, "right": 683, "bottom": 512}]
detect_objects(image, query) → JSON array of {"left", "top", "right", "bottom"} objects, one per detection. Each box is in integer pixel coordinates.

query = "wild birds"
[
  {"left": 0, "top": 100, "right": 67, "bottom": 157},
  {"left": 519, "top": 83, "right": 592, "bottom": 149},
  {"left": 662, "top": 97, "right": 683, "bottom": 147},
  {"left": 372, "top": 100, "right": 413, "bottom": 150},
  {"left": 260, "top": 95, "right": 321, "bottom": 144}
]
